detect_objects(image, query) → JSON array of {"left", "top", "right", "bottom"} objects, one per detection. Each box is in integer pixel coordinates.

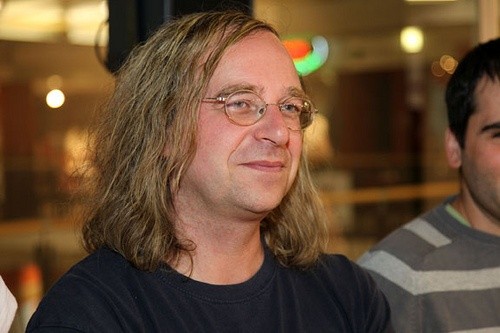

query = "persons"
[
  {"left": 356, "top": 37, "right": 500, "bottom": 333},
  {"left": 25, "top": 12, "right": 392, "bottom": 333}
]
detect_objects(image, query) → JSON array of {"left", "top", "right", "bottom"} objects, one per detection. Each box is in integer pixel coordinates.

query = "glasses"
[{"left": 202, "top": 90, "right": 318, "bottom": 131}]
